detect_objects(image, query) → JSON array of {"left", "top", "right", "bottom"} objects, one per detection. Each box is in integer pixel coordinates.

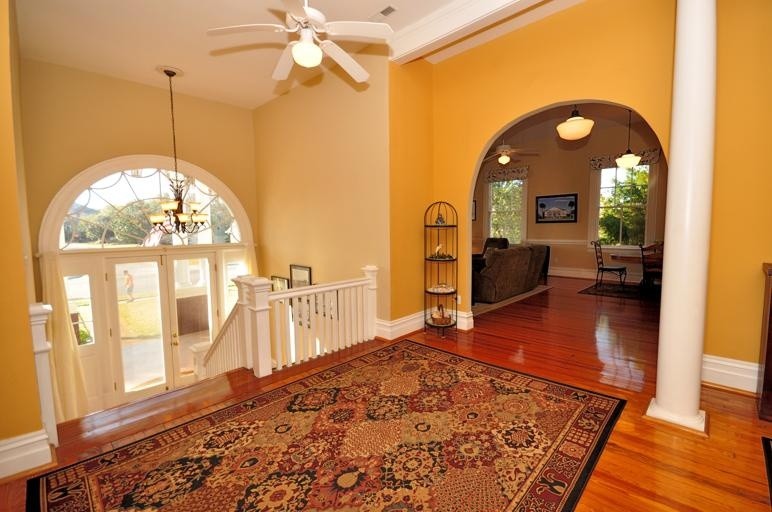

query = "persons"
[{"left": 122, "top": 270, "right": 135, "bottom": 304}]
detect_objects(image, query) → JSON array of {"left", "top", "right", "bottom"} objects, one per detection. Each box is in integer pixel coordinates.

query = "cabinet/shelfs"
[
  {"left": 756, "top": 262, "right": 772, "bottom": 421},
  {"left": 424, "top": 202, "right": 458, "bottom": 338}
]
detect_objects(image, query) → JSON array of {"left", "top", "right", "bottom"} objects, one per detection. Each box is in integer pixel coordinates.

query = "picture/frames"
[
  {"left": 536, "top": 194, "right": 577, "bottom": 223},
  {"left": 270, "top": 265, "right": 339, "bottom": 329},
  {"left": 472, "top": 200, "right": 476, "bottom": 222}
]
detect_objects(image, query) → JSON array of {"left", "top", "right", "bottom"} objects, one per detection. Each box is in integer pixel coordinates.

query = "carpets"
[
  {"left": 761, "top": 436, "right": 772, "bottom": 512},
  {"left": 472, "top": 284, "right": 552, "bottom": 317},
  {"left": 26, "top": 339, "right": 627, "bottom": 512},
  {"left": 578, "top": 283, "right": 660, "bottom": 304}
]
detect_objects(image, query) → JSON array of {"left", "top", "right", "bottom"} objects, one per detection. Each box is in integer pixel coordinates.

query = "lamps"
[
  {"left": 145, "top": 64, "right": 208, "bottom": 234},
  {"left": 615, "top": 110, "right": 642, "bottom": 169},
  {"left": 496, "top": 152, "right": 511, "bottom": 164},
  {"left": 556, "top": 104, "right": 595, "bottom": 142},
  {"left": 291, "top": 28, "right": 324, "bottom": 68}
]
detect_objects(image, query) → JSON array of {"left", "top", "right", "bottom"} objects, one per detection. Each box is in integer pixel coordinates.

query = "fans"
[
  {"left": 482, "top": 135, "right": 540, "bottom": 164},
  {"left": 205, "top": 0, "right": 393, "bottom": 83}
]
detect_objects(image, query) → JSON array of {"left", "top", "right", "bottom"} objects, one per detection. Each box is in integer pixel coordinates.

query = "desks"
[{"left": 609, "top": 254, "right": 663, "bottom": 291}]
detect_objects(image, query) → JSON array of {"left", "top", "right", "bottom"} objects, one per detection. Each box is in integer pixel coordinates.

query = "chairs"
[
  {"left": 640, "top": 242, "right": 663, "bottom": 299},
  {"left": 472, "top": 246, "right": 530, "bottom": 304},
  {"left": 481, "top": 238, "right": 509, "bottom": 257},
  {"left": 590, "top": 240, "right": 627, "bottom": 292},
  {"left": 524, "top": 245, "right": 547, "bottom": 293}
]
